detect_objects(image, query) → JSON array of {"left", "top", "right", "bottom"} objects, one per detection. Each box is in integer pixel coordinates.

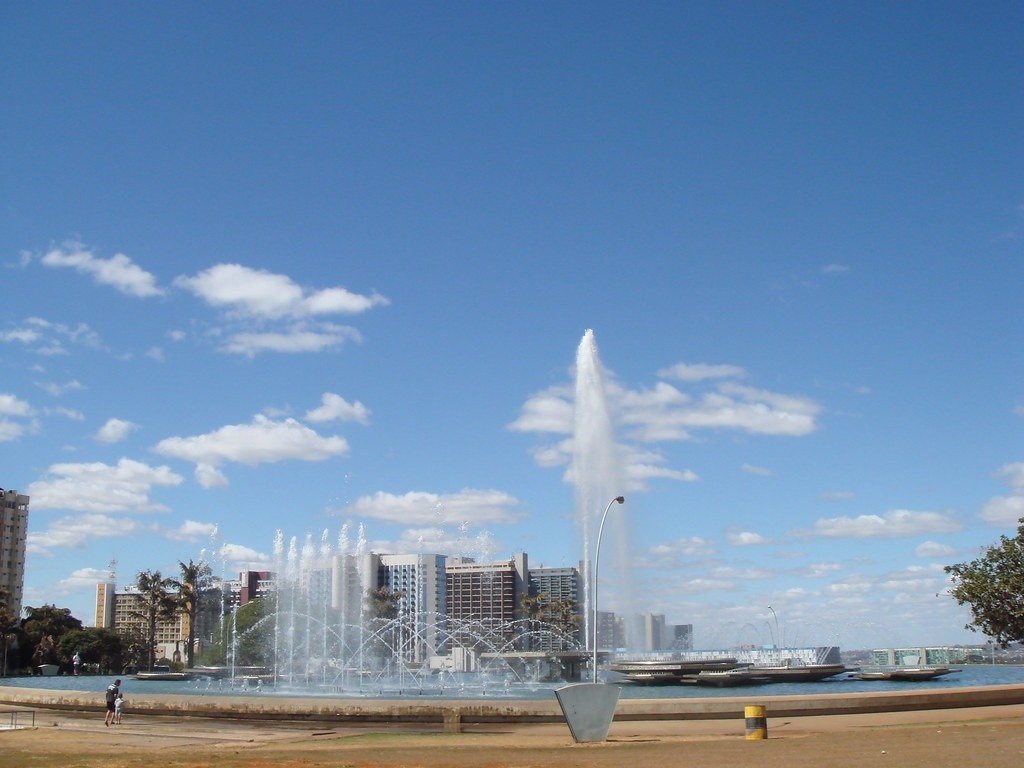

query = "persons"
[
  {"left": 105, "top": 679, "right": 121, "bottom": 727},
  {"left": 32, "top": 652, "right": 39, "bottom": 675},
  {"left": 114, "top": 694, "right": 124, "bottom": 725},
  {"left": 72, "top": 652, "right": 80, "bottom": 675}
]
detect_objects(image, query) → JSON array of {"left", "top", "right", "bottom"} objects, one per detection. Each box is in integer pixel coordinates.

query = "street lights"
[
  {"left": 594, "top": 496, "right": 625, "bottom": 681},
  {"left": 768, "top": 605, "right": 781, "bottom": 667},
  {"left": 988, "top": 643, "right": 995, "bottom": 665}
]
